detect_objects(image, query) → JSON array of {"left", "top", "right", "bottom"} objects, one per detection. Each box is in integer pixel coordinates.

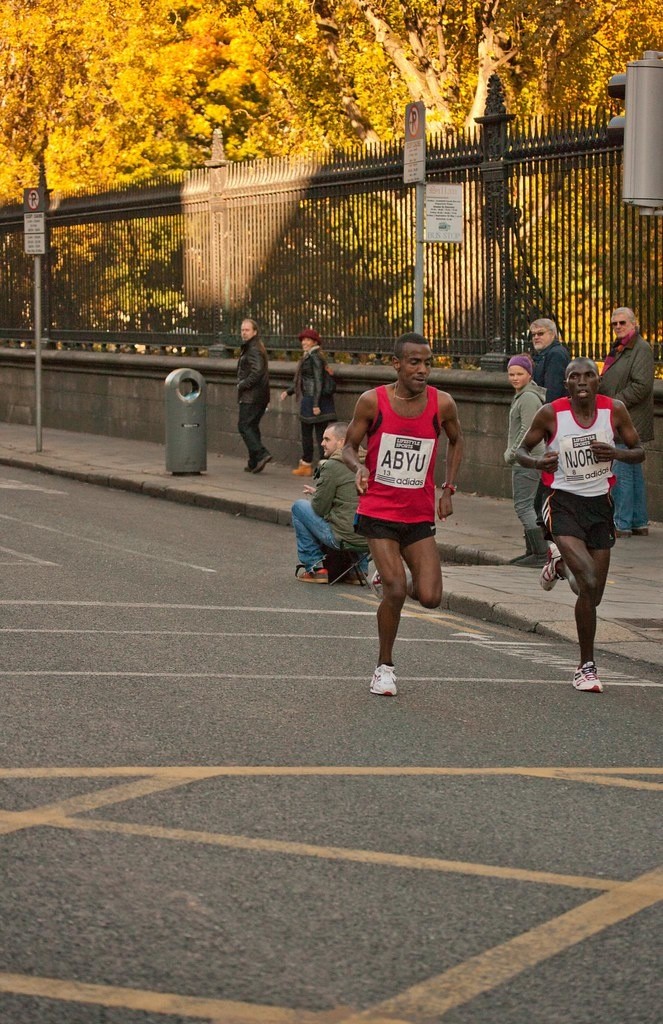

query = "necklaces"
[{"left": 394, "top": 380, "right": 420, "bottom": 400}]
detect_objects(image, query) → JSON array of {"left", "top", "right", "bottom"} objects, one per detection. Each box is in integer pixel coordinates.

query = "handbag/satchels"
[{"left": 310, "top": 352, "right": 336, "bottom": 398}]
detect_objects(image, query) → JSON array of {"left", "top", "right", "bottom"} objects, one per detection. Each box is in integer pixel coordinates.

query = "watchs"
[{"left": 441, "top": 482, "right": 456, "bottom": 495}]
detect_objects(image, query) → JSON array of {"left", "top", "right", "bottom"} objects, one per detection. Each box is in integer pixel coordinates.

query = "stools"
[{"left": 329, "top": 539, "right": 373, "bottom": 586}]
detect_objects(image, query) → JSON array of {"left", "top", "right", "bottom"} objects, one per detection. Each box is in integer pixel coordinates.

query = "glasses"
[
  {"left": 613, "top": 321, "right": 626, "bottom": 326},
  {"left": 532, "top": 330, "right": 551, "bottom": 337}
]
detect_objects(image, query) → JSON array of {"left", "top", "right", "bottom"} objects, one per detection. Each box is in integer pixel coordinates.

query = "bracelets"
[{"left": 534, "top": 459, "right": 537, "bottom": 469}]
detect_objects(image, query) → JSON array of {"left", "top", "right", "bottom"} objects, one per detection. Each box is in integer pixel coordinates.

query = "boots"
[
  {"left": 510, "top": 527, "right": 548, "bottom": 568},
  {"left": 292, "top": 460, "right": 312, "bottom": 477}
]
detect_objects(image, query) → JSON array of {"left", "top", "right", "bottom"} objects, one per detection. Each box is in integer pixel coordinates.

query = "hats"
[{"left": 298, "top": 330, "right": 322, "bottom": 345}]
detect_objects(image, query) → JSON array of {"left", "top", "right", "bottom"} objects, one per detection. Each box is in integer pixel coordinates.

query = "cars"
[{"left": 478, "top": 327, "right": 538, "bottom": 373}]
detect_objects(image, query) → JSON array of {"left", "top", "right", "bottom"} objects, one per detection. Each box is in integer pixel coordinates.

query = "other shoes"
[
  {"left": 615, "top": 529, "right": 632, "bottom": 539},
  {"left": 632, "top": 527, "right": 648, "bottom": 535},
  {"left": 244, "top": 455, "right": 272, "bottom": 473}
]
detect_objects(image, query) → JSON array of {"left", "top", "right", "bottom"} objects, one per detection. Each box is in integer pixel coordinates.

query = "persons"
[
  {"left": 503, "top": 359, "right": 551, "bottom": 568},
  {"left": 343, "top": 333, "right": 463, "bottom": 696},
  {"left": 596, "top": 306, "right": 654, "bottom": 538},
  {"left": 291, "top": 422, "right": 373, "bottom": 585},
  {"left": 280, "top": 329, "right": 338, "bottom": 476},
  {"left": 516, "top": 357, "right": 646, "bottom": 693},
  {"left": 235, "top": 320, "right": 273, "bottom": 474},
  {"left": 527, "top": 318, "right": 573, "bottom": 538}
]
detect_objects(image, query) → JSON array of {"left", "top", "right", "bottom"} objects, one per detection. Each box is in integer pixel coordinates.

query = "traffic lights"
[{"left": 604, "top": 67, "right": 629, "bottom": 144}]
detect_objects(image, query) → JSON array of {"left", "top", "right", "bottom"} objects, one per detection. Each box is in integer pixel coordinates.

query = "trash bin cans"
[{"left": 161, "top": 364, "right": 208, "bottom": 475}]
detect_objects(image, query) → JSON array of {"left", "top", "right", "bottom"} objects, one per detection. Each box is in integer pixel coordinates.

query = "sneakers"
[
  {"left": 370, "top": 664, "right": 398, "bottom": 696},
  {"left": 573, "top": 661, "right": 603, "bottom": 693},
  {"left": 371, "top": 568, "right": 384, "bottom": 599},
  {"left": 539, "top": 544, "right": 566, "bottom": 591},
  {"left": 297, "top": 568, "right": 328, "bottom": 583},
  {"left": 342, "top": 573, "right": 368, "bottom": 585}
]
